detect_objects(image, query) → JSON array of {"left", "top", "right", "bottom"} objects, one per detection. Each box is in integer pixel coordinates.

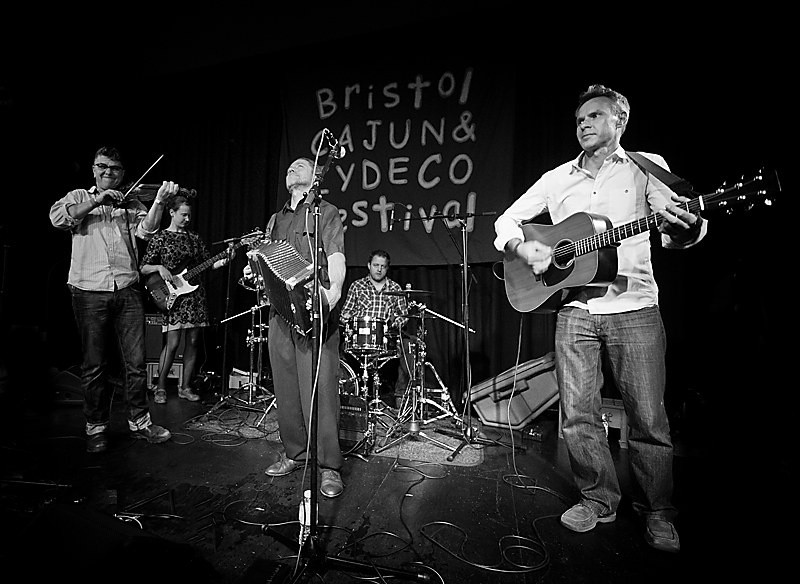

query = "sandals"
[
  {"left": 154, "top": 389, "right": 167, "bottom": 403},
  {"left": 178, "top": 385, "right": 200, "bottom": 401}
]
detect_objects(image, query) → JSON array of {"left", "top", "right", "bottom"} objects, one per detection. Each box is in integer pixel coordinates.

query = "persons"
[
  {"left": 493, "top": 85, "right": 707, "bottom": 552},
  {"left": 139, "top": 190, "right": 236, "bottom": 403},
  {"left": 338, "top": 250, "right": 412, "bottom": 410},
  {"left": 244, "top": 157, "right": 346, "bottom": 497},
  {"left": 49, "top": 146, "right": 179, "bottom": 453}
]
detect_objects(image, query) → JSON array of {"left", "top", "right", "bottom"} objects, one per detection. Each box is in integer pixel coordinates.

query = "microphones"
[
  {"left": 389, "top": 204, "right": 395, "bottom": 231},
  {"left": 325, "top": 128, "right": 346, "bottom": 159}
]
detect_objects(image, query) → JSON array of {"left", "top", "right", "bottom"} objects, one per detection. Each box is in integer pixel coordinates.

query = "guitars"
[
  {"left": 147, "top": 235, "right": 268, "bottom": 311},
  {"left": 502, "top": 164, "right": 786, "bottom": 315}
]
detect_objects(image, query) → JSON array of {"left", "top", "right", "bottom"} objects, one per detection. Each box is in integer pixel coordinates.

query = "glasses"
[{"left": 95, "top": 163, "right": 124, "bottom": 171}]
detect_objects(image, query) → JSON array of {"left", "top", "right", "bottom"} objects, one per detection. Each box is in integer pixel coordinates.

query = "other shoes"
[
  {"left": 85, "top": 422, "right": 109, "bottom": 453},
  {"left": 128, "top": 412, "right": 171, "bottom": 444}
]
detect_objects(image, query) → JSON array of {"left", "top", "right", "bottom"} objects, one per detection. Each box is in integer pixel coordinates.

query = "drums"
[
  {"left": 337, "top": 351, "right": 370, "bottom": 395},
  {"left": 342, "top": 316, "right": 390, "bottom": 357}
]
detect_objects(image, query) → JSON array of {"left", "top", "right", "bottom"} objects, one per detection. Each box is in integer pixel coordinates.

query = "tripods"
[
  {"left": 375, "top": 212, "right": 527, "bottom": 463},
  {"left": 259, "top": 150, "right": 431, "bottom": 584},
  {"left": 203, "top": 231, "right": 276, "bottom": 423}
]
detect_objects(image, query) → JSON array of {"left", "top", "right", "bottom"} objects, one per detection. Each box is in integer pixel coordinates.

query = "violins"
[{"left": 116, "top": 178, "right": 197, "bottom": 206}]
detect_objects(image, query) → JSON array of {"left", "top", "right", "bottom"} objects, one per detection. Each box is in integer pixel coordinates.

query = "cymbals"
[
  {"left": 381, "top": 290, "right": 435, "bottom": 298},
  {"left": 396, "top": 315, "right": 438, "bottom": 319}
]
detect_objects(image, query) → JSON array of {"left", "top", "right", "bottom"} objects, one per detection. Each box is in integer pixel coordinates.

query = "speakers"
[
  {"left": 144, "top": 314, "right": 185, "bottom": 364},
  {"left": 463, "top": 352, "right": 560, "bottom": 431}
]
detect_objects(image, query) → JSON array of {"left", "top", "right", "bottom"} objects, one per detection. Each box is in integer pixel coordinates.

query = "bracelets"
[{"left": 155, "top": 199, "right": 166, "bottom": 205}]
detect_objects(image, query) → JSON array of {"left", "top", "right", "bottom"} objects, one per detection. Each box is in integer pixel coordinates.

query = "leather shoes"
[
  {"left": 645, "top": 509, "right": 680, "bottom": 551},
  {"left": 561, "top": 503, "right": 616, "bottom": 532},
  {"left": 264, "top": 457, "right": 311, "bottom": 477},
  {"left": 320, "top": 471, "right": 343, "bottom": 498}
]
